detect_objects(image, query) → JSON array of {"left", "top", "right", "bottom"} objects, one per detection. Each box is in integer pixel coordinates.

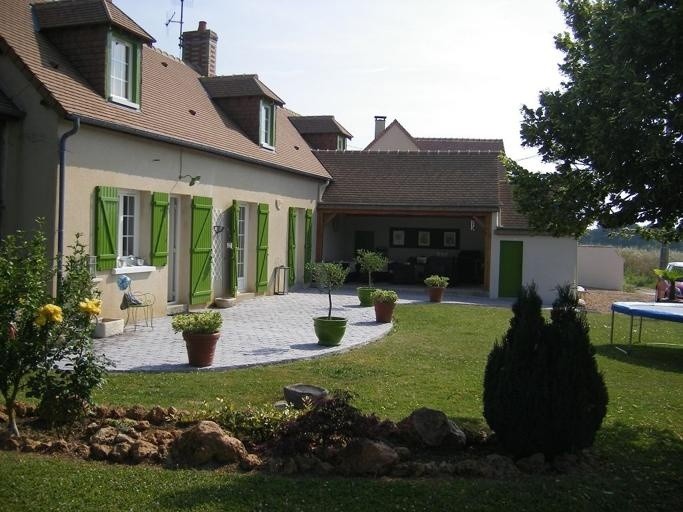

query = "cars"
[{"left": 654, "top": 260, "right": 682, "bottom": 303}]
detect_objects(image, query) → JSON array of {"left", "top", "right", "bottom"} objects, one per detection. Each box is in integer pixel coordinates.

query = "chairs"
[{"left": 122, "top": 276, "right": 156, "bottom": 331}]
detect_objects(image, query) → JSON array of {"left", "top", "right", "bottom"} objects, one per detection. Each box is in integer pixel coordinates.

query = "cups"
[{"left": 118, "top": 255, "right": 144, "bottom": 267}]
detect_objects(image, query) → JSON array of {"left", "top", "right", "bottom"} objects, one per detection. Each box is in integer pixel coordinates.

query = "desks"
[{"left": 608, "top": 300, "right": 683, "bottom": 357}]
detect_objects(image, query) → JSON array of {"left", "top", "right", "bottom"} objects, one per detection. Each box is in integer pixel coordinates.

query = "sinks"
[{"left": 89, "top": 318, "right": 124, "bottom": 338}]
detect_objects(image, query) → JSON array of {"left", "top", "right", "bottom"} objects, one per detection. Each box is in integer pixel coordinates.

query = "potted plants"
[
  {"left": 354, "top": 248, "right": 398, "bottom": 325},
  {"left": 423, "top": 273, "right": 450, "bottom": 302},
  {"left": 170, "top": 309, "right": 224, "bottom": 368},
  {"left": 304, "top": 259, "right": 353, "bottom": 348}
]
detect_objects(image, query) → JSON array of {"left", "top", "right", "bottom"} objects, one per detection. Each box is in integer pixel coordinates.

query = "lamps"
[{"left": 178, "top": 173, "right": 201, "bottom": 187}]
